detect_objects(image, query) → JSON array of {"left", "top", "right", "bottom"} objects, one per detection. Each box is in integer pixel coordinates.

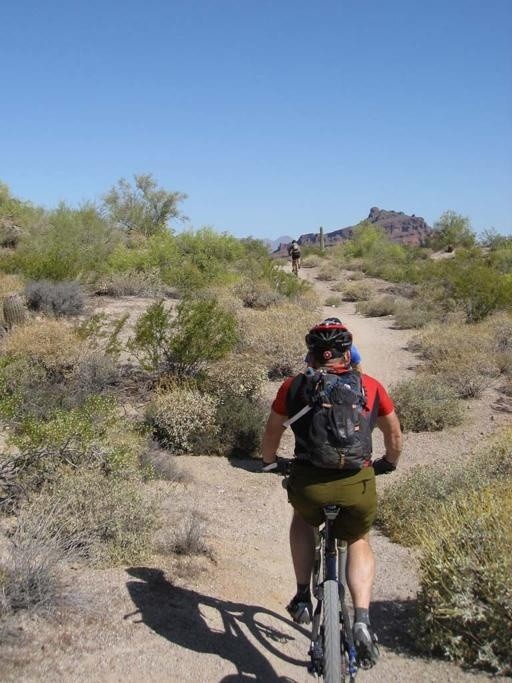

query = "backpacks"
[{"left": 304, "top": 367, "right": 373, "bottom": 470}]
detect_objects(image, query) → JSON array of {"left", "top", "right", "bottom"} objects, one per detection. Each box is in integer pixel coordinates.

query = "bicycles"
[
  {"left": 289, "top": 252, "right": 301, "bottom": 277},
  {"left": 257, "top": 442, "right": 392, "bottom": 683}
]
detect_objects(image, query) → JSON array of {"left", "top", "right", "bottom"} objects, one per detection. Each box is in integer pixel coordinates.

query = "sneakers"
[
  {"left": 353, "top": 621, "right": 380, "bottom": 665},
  {"left": 286, "top": 596, "right": 313, "bottom": 625}
]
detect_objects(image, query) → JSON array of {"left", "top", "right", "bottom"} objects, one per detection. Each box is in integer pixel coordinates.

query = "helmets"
[{"left": 305, "top": 318, "right": 353, "bottom": 360}]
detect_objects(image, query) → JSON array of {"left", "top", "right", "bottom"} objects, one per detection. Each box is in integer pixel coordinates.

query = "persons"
[{"left": 288, "top": 240, "right": 301, "bottom": 272}]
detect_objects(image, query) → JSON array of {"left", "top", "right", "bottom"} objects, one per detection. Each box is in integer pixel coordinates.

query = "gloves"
[
  {"left": 372, "top": 455, "right": 396, "bottom": 475},
  {"left": 261, "top": 456, "right": 293, "bottom": 475}
]
